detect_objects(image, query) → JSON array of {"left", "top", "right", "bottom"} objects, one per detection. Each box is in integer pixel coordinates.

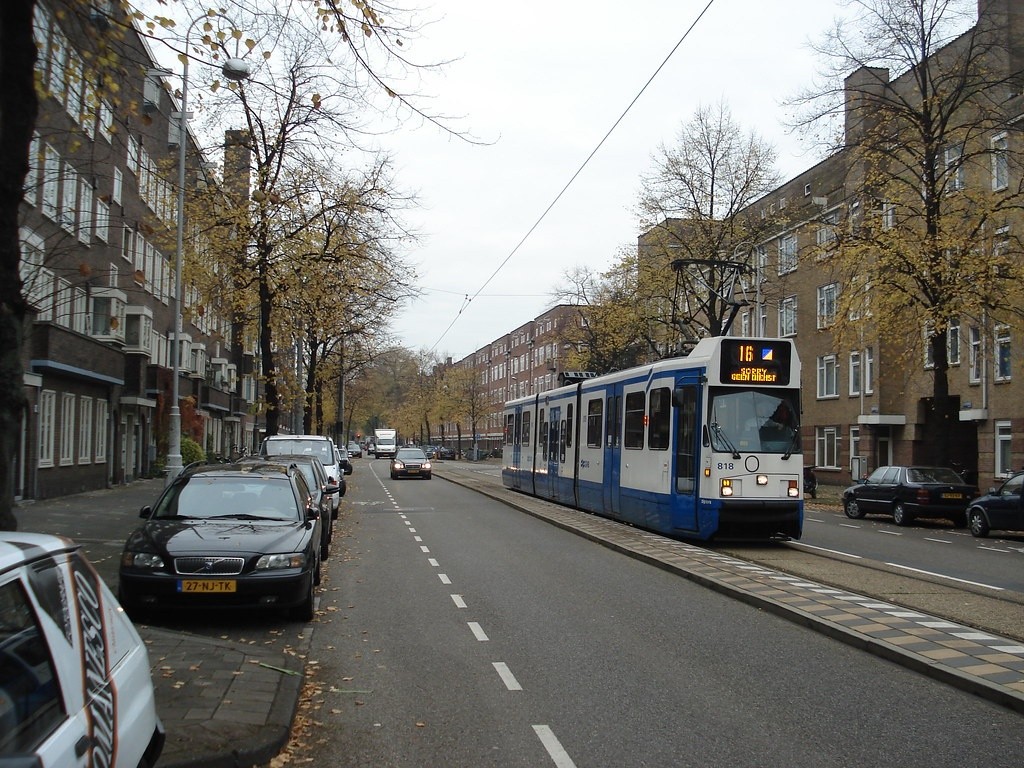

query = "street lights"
[{"left": 164, "top": 11, "right": 250, "bottom": 479}]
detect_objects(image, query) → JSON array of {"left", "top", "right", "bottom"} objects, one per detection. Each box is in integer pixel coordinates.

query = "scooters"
[{"left": 802, "top": 463, "right": 818, "bottom": 499}]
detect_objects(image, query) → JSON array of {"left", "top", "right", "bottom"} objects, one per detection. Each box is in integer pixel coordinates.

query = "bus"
[{"left": 502, "top": 335, "right": 806, "bottom": 544}]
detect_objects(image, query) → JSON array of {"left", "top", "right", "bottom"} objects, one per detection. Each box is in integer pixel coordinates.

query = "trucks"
[{"left": 374, "top": 428, "right": 397, "bottom": 459}]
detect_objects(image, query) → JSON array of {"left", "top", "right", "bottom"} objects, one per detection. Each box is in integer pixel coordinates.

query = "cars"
[
  {"left": 238, "top": 453, "right": 340, "bottom": 561},
  {"left": 966, "top": 471, "right": 1024, "bottom": 537},
  {"left": 397, "top": 441, "right": 503, "bottom": 461},
  {"left": 333, "top": 439, "right": 376, "bottom": 497},
  {"left": 843, "top": 465, "right": 979, "bottom": 528},
  {"left": 388, "top": 448, "right": 433, "bottom": 480},
  {"left": 0, "top": 528, "right": 169, "bottom": 768}
]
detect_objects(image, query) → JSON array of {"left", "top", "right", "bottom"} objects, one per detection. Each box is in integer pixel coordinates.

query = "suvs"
[
  {"left": 258, "top": 434, "right": 340, "bottom": 522},
  {"left": 117, "top": 457, "right": 322, "bottom": 625}
]
